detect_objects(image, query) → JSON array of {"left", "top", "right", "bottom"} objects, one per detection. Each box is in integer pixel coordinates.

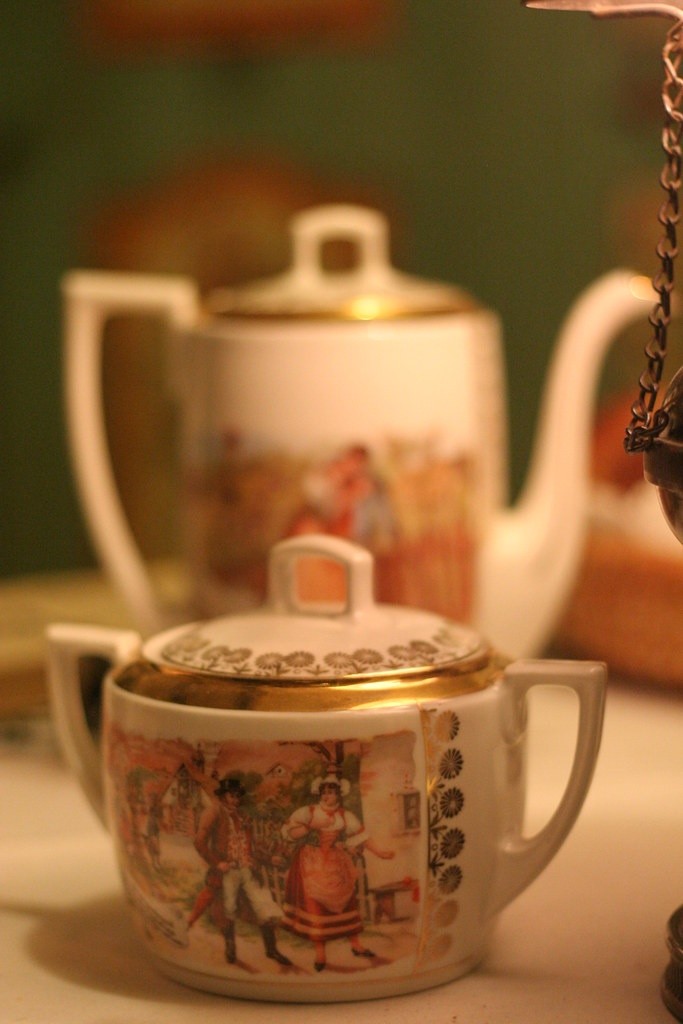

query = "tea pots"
[{"left": 58, "top": 204, "right": 671, "bottom": 671}]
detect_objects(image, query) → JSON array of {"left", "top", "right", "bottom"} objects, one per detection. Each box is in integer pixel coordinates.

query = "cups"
[{"left": 46, "top": 533, "right": 608, "bottom": 1004}]
